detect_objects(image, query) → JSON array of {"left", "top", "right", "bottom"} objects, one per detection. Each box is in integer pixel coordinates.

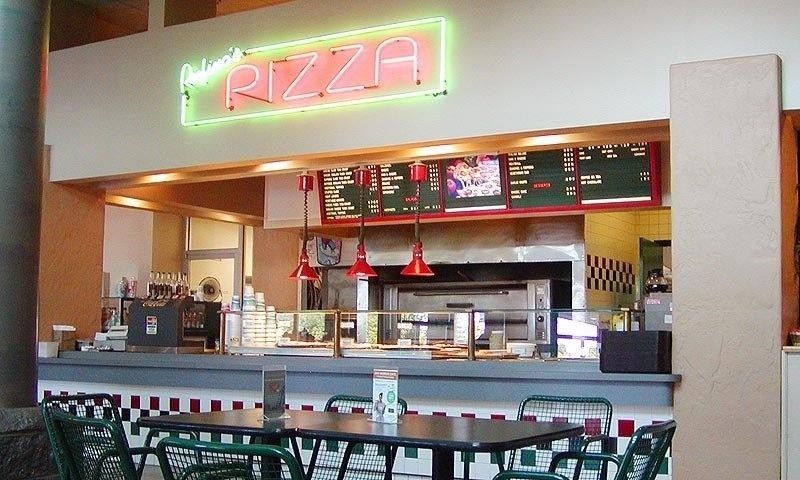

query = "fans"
[{"left": 200, "top": 277, "right": 223, "bottom": 304}]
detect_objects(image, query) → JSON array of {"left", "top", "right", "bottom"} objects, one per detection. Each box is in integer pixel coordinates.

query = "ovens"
[{"left": 394, "top": 287, "right": 530, "bottom": 340}]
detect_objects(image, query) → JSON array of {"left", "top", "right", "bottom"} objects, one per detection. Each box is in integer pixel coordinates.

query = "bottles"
[
  {"left": 489, "top": 331, "right": 507, "bottom": 350},
  {"left": 117, "top": 276, "right": 137, "bottom": 298},
  {"left": 215, "top": 339, "right": 220, "bottom": 355},
  {"left": 185, "top": 308, "right": 207, "bottom": 329},
  {"left": 147, "top": 271, "right": 190, "bottom": 298}
]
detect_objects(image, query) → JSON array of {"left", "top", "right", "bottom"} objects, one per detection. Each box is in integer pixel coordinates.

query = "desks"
[{"left": 129, "top": 403, "right": 584, "bottom": 479}]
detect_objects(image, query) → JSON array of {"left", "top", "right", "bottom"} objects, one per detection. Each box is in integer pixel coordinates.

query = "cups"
[
  {"left": 197, "top": 285, "right": 204, "bottom": 300},
  {"left": 397, "top": 339, "right": 411, "bottom": 347},
  {"left": 225, "top": 286, "right": 289, "bottom": 346}
]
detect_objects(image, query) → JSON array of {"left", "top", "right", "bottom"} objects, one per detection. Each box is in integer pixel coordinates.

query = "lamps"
[
  {"left": 344, "top": 165, "right": 380, "bottom": 279},
  {"left": 284, "top": 171, "right": 325, "bottom": 282},
  {"left": 398, "top": 157, "right": 438, "bottom": 277}
]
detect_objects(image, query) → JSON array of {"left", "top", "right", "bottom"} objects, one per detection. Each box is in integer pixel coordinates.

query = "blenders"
[{"left": 53, "top": 324, "right": 76, "bottom": 352}]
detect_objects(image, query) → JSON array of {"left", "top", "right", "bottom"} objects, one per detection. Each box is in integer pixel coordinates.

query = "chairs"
[
  {"left": 40, "top": 393, "right": 171, "bottom": 478},
  {"left": 494, "top": 396, "right": 613, "bottom": 478},
  {"left": 156, "top": 436, "right": 313, "bottom": 480},
  {"left": 492, "top": 416, "right": 679, "bottom": 478},
  {"left": 286, "top": 395, "right": 408, "bottom": 479}
]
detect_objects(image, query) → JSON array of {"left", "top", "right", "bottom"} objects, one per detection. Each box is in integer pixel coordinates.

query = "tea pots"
[{"left": 644, "top": 269, "right": 669, "bottom": 292}]
